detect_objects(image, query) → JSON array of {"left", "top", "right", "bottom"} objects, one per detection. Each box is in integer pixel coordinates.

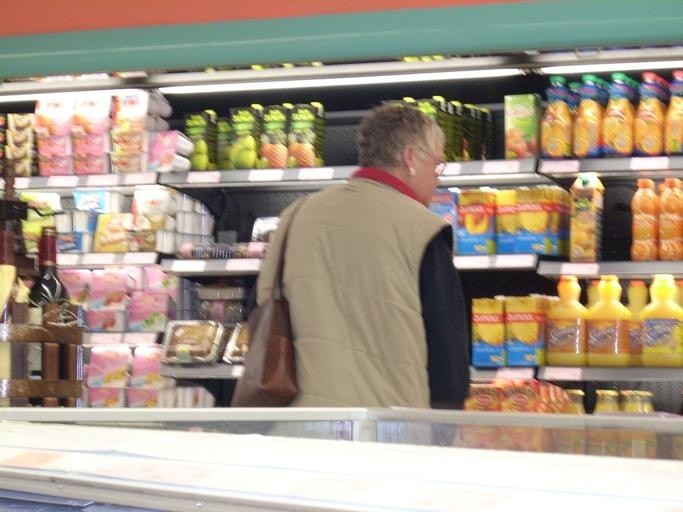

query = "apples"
[
  {"left": 193, "top": 134, "right": 207, "bottom": 169},
  {"left": 222, "top": 135, "right": 258, "bottom": 169}
]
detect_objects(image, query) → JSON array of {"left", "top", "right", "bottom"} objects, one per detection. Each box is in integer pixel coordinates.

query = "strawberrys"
[
  {"left": 290, "top": 137, "right": 315, "bottom": 168},
  {"left": 260, "top": 138, "right": 286, "bottom": 169}
]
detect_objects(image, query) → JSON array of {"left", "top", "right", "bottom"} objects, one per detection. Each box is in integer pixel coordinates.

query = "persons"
[{"left": 240, "top": 102, "right": 472, "bottom": 410}]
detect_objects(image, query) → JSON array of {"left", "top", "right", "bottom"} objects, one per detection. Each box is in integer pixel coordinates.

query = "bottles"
[
  {"left": 601, "top": 73, "right": 633, "bottom": 158},
  {"left": 573, "top": 74, "right": 600, "bottom": 159},
  {"left": 27, "top": 227, "right": 70, "bottom": 408},
  {"left": 627, "top": 78, "right": 639, "bottom": 105},
  {"left": 621, "top": 390, "right": 645, "bottom": 457},
  {"left": 663, "top": 71, "right": 683, "bottom": 156},
  {"left": 628, "top": 179, "right": 656, "bottom": 261},
  {"left": 568, "top": 81, "right": 582, "bottom": 109},
  {"left": 548, "top": 274, "right": 683, "bottom": 367},
  {"left": 597, "top": 80, "right": 609, "bottom": 107},
  {"left": 633, "top": 72, "right": 662, "bottom": 157},
  {"left": 658, "top": 178, "right": 683, "bottom": 261},
  {"left": 541, "top": 76, "right": 571, "bottom": 159},
  {"left": 641, "top": 392, "right": 655, "bottom": 458},
  {"left": 590, "top": 389, "right": 618, "bottom": 456},
  {"left": 558, "top": 389, "right": 584, "bottom": 454},
  {"left": 0, "top": 231, "right": 24, "bottom": 407},
  {"left": 658, "top": 76, "right": 672, "bottom": 109}
]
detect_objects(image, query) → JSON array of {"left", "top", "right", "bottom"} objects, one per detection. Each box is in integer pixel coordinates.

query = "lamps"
[
  {"left": 526, "top": 48, "right": 683, "bottom": 76},
  {"left": 1, "top": 77, "right": 155, "bottom": 104},
  {"left": 147, "top": 55, "right": 533, "bottom": 97}
]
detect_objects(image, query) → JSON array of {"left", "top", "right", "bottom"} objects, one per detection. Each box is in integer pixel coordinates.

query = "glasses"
[{"left": 419, "top": 147, "right": 447, "bottom": 173}]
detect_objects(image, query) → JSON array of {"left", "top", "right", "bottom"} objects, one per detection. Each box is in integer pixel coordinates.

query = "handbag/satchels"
[{"left": 231, "top": 191, "right": 306, "bottom": 406}]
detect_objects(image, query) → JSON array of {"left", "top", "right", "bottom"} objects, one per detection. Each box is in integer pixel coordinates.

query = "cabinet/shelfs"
[
  {"left": 0, "top": 68, "right": 681, "bottom": 460},
  {"left": 0, "top": 152, "right": 85, "bottom": 410}
]
